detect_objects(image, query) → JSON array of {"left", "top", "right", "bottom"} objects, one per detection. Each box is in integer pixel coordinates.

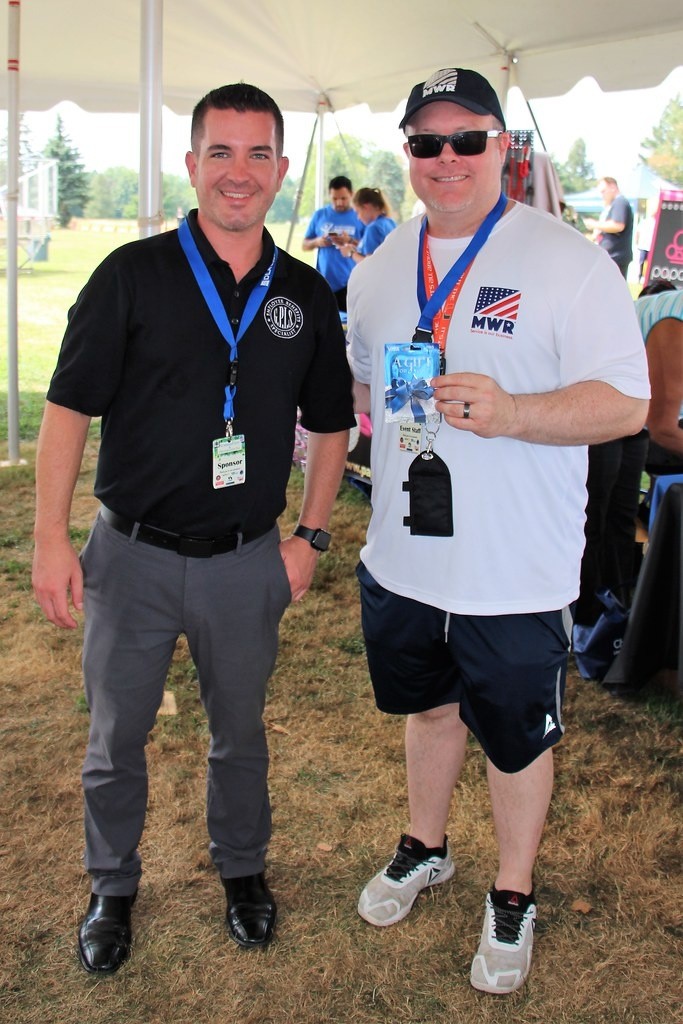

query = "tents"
[{"left": 560, "top": 160, "right": 680, "bottom": 214}]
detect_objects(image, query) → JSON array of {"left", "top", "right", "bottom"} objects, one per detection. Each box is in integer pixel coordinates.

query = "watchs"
[{"left": 294, "top": 525, "right": 332, "bottom": 552}]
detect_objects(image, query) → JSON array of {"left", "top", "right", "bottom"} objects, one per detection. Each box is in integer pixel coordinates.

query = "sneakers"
[
  {"left": 357, "top": 833, "right": 455, "bottom": 926},
  {"left": 469, "top": 889, "right": 535, "bottom": 993}
]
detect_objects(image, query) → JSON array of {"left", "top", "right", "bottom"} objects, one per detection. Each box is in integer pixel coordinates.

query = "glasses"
[{"left": 408, "top": 130, "right": 501, "bottom": 159}]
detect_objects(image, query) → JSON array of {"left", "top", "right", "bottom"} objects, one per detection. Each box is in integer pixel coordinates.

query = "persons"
[
  {"left": 338, "top": 188, "right": 397, "bottom": 266},
  {"left": 346, "top": 67, "right": 652, "bottom": 993},
  {"left": 301, "top": 176, "right": 364, "bottom": 315},
  {"left": 567, "top": 281, "right": 683, "bottom": 622},
  {"left": 32, "top": 84, "right": 358, "bottom": 973},
  {"left": 635, "top": 212, "right": 656, "bottom": 283},
  {"left": 583, "top": 176, "right": 633, "bottom": 282}
]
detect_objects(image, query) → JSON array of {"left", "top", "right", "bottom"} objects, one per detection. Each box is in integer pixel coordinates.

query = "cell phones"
[
  {"left": 328, "top": 232, "right": 338, "bottom": 237},
  {"left": 335, "top": 240, "right": 344, "bottom": 246}
]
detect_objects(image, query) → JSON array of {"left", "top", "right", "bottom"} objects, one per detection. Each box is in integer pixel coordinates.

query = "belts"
[{"left": 100, "top": 503, "right": 275, "bottom": 558}]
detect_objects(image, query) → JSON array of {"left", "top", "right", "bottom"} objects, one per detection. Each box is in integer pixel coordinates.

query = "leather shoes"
[
  {"left": 221, "top": 870, "right": 276, "bottom": 947},
  {"left": 78, "top": 887, "right": 139, "bottom": 975}
]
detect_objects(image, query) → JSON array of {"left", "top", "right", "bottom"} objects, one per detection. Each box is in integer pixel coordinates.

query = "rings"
[{"left": 464, "top": 404, "right": 470, "bottom": 417}]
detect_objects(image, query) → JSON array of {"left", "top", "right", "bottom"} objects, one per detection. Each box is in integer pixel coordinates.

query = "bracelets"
[
  {"left": 348, "top": 251, "right": 354, "bottom": 257},
  {"left": 349, "top": 237, "right": 355, "bottom": 243}
]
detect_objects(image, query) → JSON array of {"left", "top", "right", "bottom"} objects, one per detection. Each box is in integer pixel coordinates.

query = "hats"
[{"left": 398, "top": 67, "right": 506, "bottom": 132}]
